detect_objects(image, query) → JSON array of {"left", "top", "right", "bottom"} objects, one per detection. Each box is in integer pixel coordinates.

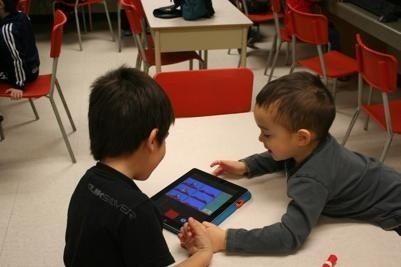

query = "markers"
[{"left": 321, "top": 253, "right": 339, "bottom": 267}]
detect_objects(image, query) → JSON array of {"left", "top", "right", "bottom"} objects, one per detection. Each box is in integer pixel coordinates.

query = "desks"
[{"left": 141, "top": 0, "right": 254, "bottom": 76}]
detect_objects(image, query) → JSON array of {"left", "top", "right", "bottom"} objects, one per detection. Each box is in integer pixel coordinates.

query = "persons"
[
  {"left": 63, "top": 64, "right": 213, "bottom": 267},
  {"left": 0, "top": 0, "right": 41, "bottom": 121},
  {"left": 202, "top": 71, "right": 401, "bottom": 257}
]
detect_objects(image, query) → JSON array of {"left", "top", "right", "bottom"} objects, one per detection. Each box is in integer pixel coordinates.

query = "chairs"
[
  {"left": 288, "top": 3, "right": 359, "bottom": 100},
  {"left": 227, "top": 0, "right": 290, "bottom": 67},
  {"left": 263, "top": 0, "right": 332, "bottom": 84},
  {"left": 0, "top": 9, "right": 79, "bottom": 164},
  {"left": 51, "top": 0, "right": 116, "bottom": 52},
  {"left": 119, "top": 0, "right": 207, "bottom": 78},
  {"left": 340, "top": 33, "right": 401, "bottom": 164},
  {"left": 117, "top": 0, "right": 147, "bottom": 53}
]
